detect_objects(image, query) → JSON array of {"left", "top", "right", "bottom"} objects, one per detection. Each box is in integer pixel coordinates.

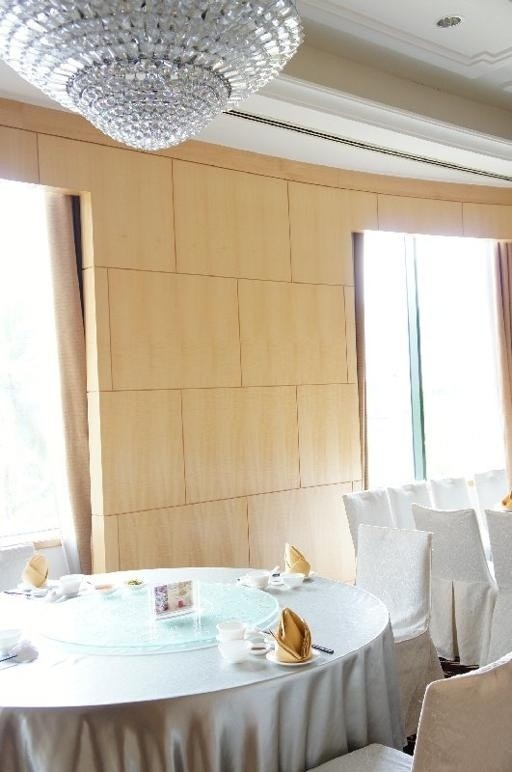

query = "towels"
[
  {"left": 23, "top": 554, "right": 50, "bottom": 588},
  {"left": 267, "top": 607, "right": 312, "bottom": 663},
  {"left": 283, "top": 542, "right": 310, "bottom": 577}
]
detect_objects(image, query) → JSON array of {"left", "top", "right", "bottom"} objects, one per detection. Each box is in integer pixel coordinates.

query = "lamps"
[{"left": 0, "top": 1, "right": 306, "bottom": 153}]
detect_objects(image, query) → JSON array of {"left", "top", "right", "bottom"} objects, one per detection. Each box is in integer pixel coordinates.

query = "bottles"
[{"left": 270, "top": 566, "right": 281, "bottom": 586}]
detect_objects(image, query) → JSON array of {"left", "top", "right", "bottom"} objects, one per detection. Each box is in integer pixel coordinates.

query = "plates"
[
  {"left": 125, "top": 575, "right": 150, "bottom": 589},
  {"left": 250, "top": 642, "right": 271, "bottom": 655},
  {"left": 265, "top": 649, "right": 321, "bottom": 667},
  {"left": 87, "top": 581, "right": 119, "bottom": 596},
  {"left": 15, "top": 578, "right": 56, "bottom": 596},
  {"left": 304, "top": 569, "right": 316, "bottom": 580}
]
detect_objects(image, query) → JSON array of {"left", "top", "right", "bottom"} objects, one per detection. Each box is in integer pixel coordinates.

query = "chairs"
[
  {"left": 353, "top": 522, "right": 446, "bottom": 737},
  {"left": 307, "top": 649, "right": 512, "bottom": 771},
  {"left": 343, "top": 468, "right": 512, "bottom": 668},
  {"left": 0, "top": 541, "right": 36, "bottom": 590}
]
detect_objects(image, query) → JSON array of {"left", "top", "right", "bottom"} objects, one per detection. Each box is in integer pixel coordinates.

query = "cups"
[
  {"left": 217, "top": 621, "right": 248, "bottom": 642},
  {"left": 247, "top": 567, "right": 270, "bottom": 589}
]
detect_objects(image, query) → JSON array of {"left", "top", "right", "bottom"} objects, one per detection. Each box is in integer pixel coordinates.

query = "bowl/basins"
[
  {"left": 59, "top": 573, "right": 84, "bottom": 595},
  {"left": 0, "top": 628, "right": 23, "bottom": 650},
  {"left": 217, "top": 640, "right": 254, "bottom": 663},
  {"left": 281, "top": 572, "right": 305, "bottom": 588}
]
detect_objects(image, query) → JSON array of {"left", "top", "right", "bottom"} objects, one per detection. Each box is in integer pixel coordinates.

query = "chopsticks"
[
  {"left": 262, "top": 628, "right": 334, "bottom": 653},
  {"left": 2, "top": 590, "right": 32, "bottom": 596},
  {"left": 0, "top": 654, "right": 18, "bottom": 662}
]
